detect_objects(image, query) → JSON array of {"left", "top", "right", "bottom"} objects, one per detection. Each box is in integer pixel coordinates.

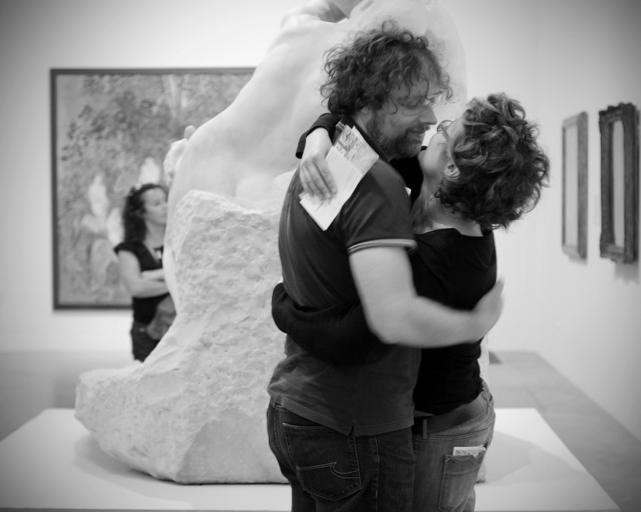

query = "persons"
[
  {"left": 263, "top": 19, "right": 507, "bottom": 510},
  {"left": 111, "top": 183, "right": 170, "bottom": 363},
  {"left": 271, "top": 93, "right": 554, "bottom": 510}
]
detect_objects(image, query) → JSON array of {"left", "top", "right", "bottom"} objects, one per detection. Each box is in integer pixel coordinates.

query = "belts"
[{"left": 412, "top": 379, "right": 490, "bottom": 435}]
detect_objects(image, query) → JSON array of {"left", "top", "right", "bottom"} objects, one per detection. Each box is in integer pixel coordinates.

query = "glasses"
[{"left": 436, "top": 119, "right": 453, "bottom": 142}]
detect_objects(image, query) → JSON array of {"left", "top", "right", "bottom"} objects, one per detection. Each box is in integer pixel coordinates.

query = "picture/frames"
[
  {"left": 561, "top": 102, "right": 639, "bottom": 265},
  {"left": 49, "top": 68, "right": 257, "bottom": 311}
]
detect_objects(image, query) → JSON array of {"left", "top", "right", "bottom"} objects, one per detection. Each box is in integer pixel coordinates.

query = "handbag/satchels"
[{"left": 144, "top": 294, "right": 175, "bottom": 341}]
